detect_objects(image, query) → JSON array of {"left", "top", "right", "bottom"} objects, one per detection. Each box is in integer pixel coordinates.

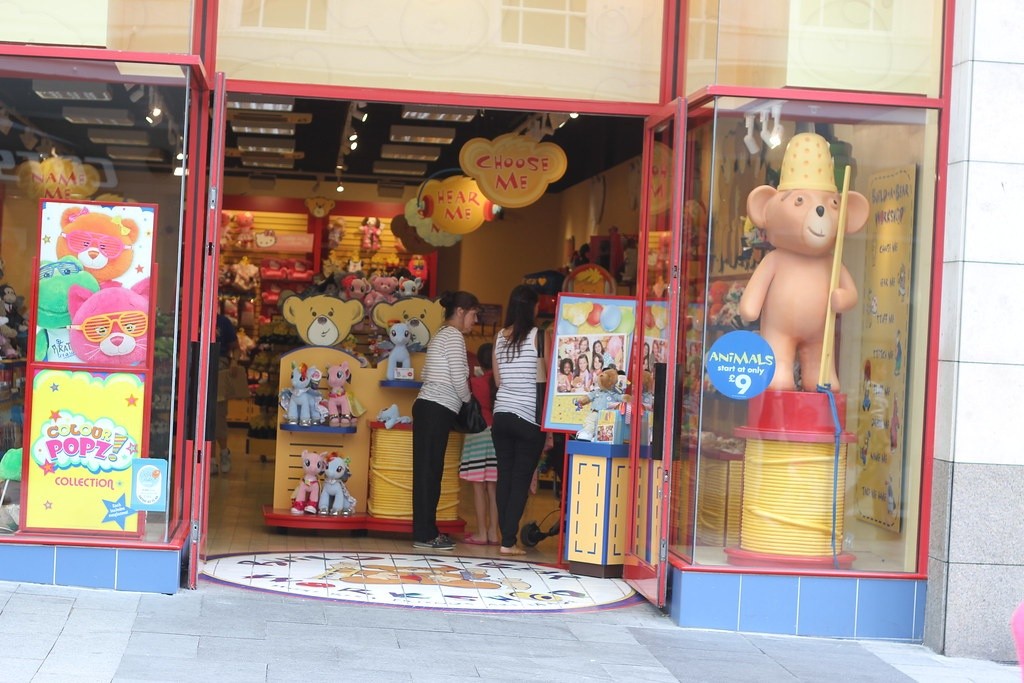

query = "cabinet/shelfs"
[{"left": 0, "top": 194, "right": 468, "bottom": 533}]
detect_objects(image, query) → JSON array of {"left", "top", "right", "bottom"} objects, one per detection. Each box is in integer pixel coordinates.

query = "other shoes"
[
  {"left": 209, "top": 464, "right": 219, "bottom": 475},
  {"left": 500, "top": 546, "right": 527, "bottom": 556},
  {"left": 221, "top": 447, "right": 232, "bottom": 472}
]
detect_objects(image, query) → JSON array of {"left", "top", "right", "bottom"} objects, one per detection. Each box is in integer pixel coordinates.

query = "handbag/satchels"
[
  {"left": 535, "top": 328, "right": 548, "bottom": 426},
  {"left": 448, "top": 398, "right": 487, "bottom": 434}
]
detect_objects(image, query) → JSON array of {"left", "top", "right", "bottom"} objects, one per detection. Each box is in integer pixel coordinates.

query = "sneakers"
[
  {"left": 413, "top": 537, "right": 453, "bottom": 550},
  {"left": 435, "top": 534, "right": 456, "bottom": 547}
]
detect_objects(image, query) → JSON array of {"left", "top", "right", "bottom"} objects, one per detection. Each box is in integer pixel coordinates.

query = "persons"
[
  {"left": 632, "top": 339, "right": 702, "bottom": 376},
  {"left": 557, "top": 337, "right": 605, "bottom": 393},
  {"left": 490, "top": 284, "right": 548, "bottom": 554},
  {"left": 458, "top": 343, "right": 499, "bottom": 546},
  {"left": 192, "top": 289, "right": 241, "bottom": 475},
  {"left": 412, "top": 291, "right": 478, "bottom": 551}
]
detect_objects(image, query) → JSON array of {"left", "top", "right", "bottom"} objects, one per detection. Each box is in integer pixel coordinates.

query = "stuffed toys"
[
  {"left": 220, "top": 211, "right": 255, "bottom": 251},
  {"left": 320, "top": 362, "right": 368, "bottom": 426},
  {"left": 377, "top": 403, "right": 411, "bottom": 429},
  {"left": 321, "top": 217, "right": 428, "bottom": 369},
  {"left": 278, "top": 361, "right": 329, "bottom": 426},
  {"left": 707, "top": 280, "right": 751, "bottom": 326},
  {"left": 641, "top": 369, "right": 653, "bottom": 415},
  {"left": 290, "top": 449, "right": 328, "bottom": 514},
  {"left": 259, "top": 258, "right": 313, "bottom": 325},
  {"left": 317, "top": 451, "right": 356, "bottom": 516},
  {"left": 0, "top": 257, "right": 29, "bottom": 359},
  {"left": 387, "top": 320, "right": 411, "bottom": 381},
  {"left": 576, "top": 365, "right": 631, "bottom": 442},
  {"left": 704, "top": 351, "right": 717, "bottom": 392},
  {"left": 149, "top": 304, "right": 181, "bottom": 460},
  {"left": 570, "top": 377, "right": 591, "bottom": 392}
]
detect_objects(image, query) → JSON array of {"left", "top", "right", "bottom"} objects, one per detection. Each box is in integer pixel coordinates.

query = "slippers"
[
  {"left": 462, "top": 537, "right": 488, "bottom": 545},
  {"left": 488, "top": 541, "right": 499, "bottom": 546}
]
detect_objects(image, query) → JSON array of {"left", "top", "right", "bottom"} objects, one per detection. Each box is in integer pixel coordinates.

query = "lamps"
[
  {"left": 345, "top": 140, "right": 358, "bottom": 150},
  {"left": 350, "top": 109, "right": 368, "bottom": 122},
  {"left": 346, "top": 127, "right": 358, "bottom": 141},
  {"left": 32, "top": 79, "right": 164, "bottom": 161},
  {"left": 226, "top": 92, "right": 295, "bottom": 112},
  {"left": 312, "top": 177, "right": 322, "bottom": 193},
  {"left": 551, "top": 113, "right": 566, "bottom": 128},
  {"left": 380, "top": 144, "right": 440, "bottom": 161},
  {"left": 231, "top": 121, "right": 294, "bottom": 135},
  {"left": 173, "top": 134, "right": 188, "bottom": 176},
  {"left": 401, "top": 104, "right": 478, "bottom": 122},
  {"left": 372, "top": 160, "right": 427, "bottom": 175},
  {"left": 167, "top": 119, "right": 175, "bottom": 146},
  {"left": 237, "top": 137, "right": 296, "bottom": 153},
  {"left": 335, "top": 157, "right": 348, "bottom": 192},
  {"left": 389, "top": 125, "right": 456, "bottom": 144},
  {"left": 242, "top": 156, "right": 294, "bottom": 169},
  {"left": 759, "top": 110, "right": 778, "bottom": 149},
  {"left": 743, "top": 115, "right": 760, "bottom": 154},
  {"left": 769, "top": 104, "right": 784, "bottom": 146}
]
